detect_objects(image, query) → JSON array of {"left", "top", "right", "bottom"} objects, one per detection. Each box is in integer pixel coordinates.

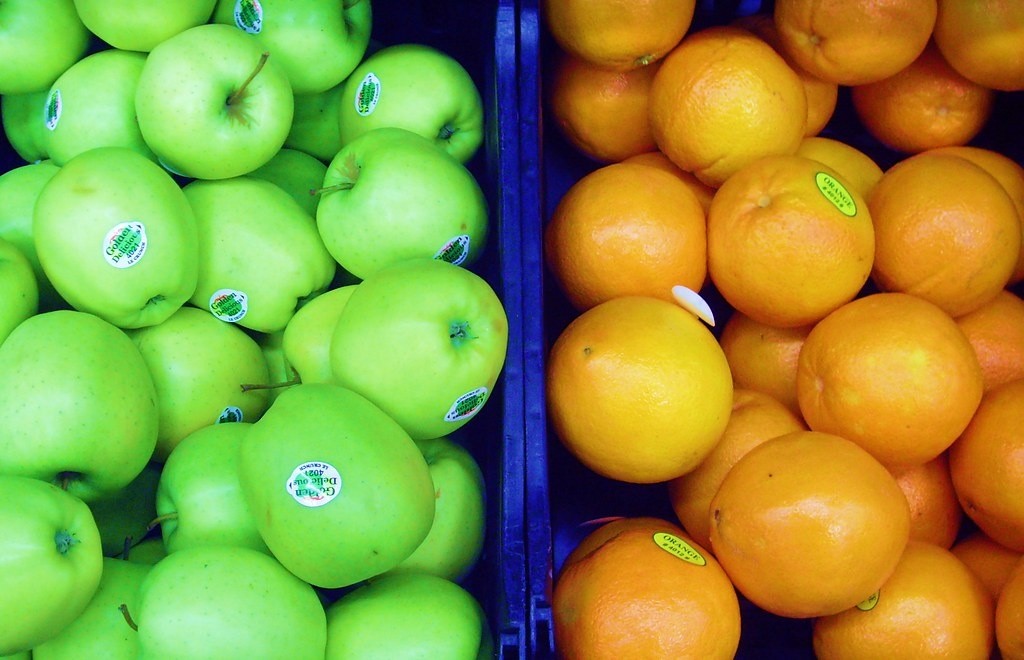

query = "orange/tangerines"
[{"left": 544, "top": 0, "right": 1024, "bottom": 660}]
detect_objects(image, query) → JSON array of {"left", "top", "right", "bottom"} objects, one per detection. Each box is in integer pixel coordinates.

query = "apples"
[{"left": 0, "top": 0, "right": 511, "bottom": 660}]
